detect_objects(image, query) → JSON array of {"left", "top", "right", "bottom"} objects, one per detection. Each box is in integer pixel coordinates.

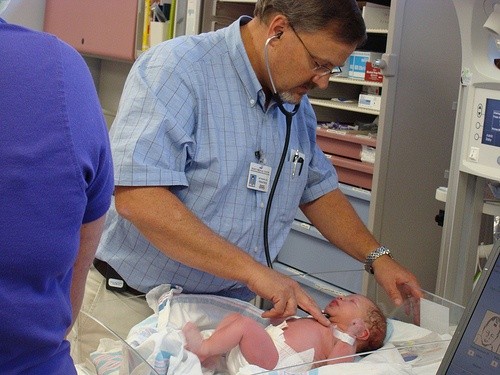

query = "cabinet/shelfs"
[{"left": 186, "top": 0, "right": 461, "bottom": 318}]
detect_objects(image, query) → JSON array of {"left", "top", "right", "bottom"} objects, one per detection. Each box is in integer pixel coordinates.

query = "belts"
[{"left": 93, "top": 258, "right": 145, "bottom": 299}]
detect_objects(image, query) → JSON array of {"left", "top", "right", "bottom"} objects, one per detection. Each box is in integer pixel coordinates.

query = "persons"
[
  {"left": 66, "top": 0, "right": 424, "bottom": 374},
  {"left": 179, "top": 294, "right": 387, "bottom": 373},
  {"left": 0, "top": 0, "right": 117, "bottom": 374}
]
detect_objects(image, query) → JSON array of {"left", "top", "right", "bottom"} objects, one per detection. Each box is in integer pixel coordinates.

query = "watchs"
[{"left": 363, "top": 245, "right": 396, "bottom": 274}]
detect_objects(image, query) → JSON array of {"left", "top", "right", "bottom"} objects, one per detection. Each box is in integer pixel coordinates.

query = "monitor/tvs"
[{"left": 437, "top": 237, "right": 500, "bottom": 375}]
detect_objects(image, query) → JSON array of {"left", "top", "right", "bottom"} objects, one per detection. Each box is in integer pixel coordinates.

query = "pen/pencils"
[
  {"left": 297, "top": 157, "right": 304, "bottom": 176},
  {"left": 292, "top": 150, "right": 299, "bottom": 175}
]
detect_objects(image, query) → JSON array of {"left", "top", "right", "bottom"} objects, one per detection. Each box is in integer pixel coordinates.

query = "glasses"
[{"left": 289, "top": 23, "right": 343, "bottom": 77}]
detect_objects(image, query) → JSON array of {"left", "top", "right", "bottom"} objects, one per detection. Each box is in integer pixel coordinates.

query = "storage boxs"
[{"left": 349, "top": 51, "right": 381, "bottom": 81}]
